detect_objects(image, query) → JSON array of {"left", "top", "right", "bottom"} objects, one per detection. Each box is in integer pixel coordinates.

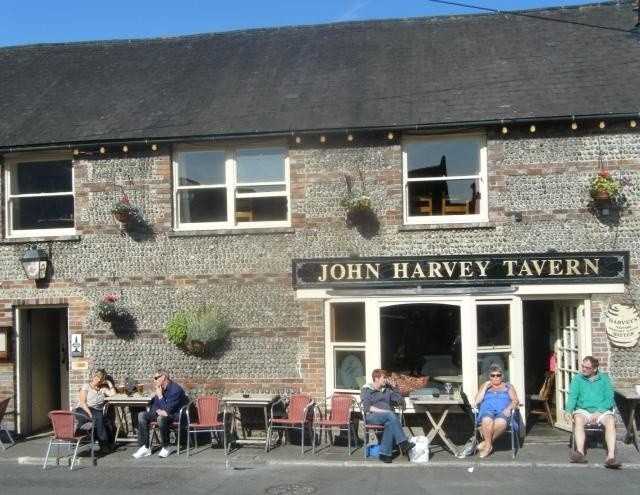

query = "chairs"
[
  {"left": 143, "top": 396, "right": 190, "bottom": 458},
  {"left": 354, "top": 397, "right": 406, "bottom": 459},
  {"left": 526, "top": 368, "right": 559, "bottom": 435},
  {"left": 473, "top": 402, "right": 522, "bottom": 459},
  {"left": 437, "top": 192, "right": 470, "bottom": 216},
  {"left": 235, "top": 211, "right": 255, "bottom": 223},
  {"left": 410, "top": 194, "right": 434, "bottom": 217},
  {"left": 39, "top": 408, "right": 94, "bottom": 475},
  {"left": 183, "top": 393, "right": 230, "bottom": 459},
  {"left": 310, "top": 393, "right": 358, "bottom": 455},
  {"left": 70, "top": 402, "right": 114, "bottom": 448},
  {"left": 0, "top": 394, "right": 19, "bottom": 455},
  {"left": 264, "top": 393, "right": 314, "bottom": 457}
]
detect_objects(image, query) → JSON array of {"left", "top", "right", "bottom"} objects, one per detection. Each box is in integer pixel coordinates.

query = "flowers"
[
  {"left": 589, "top": 171, "right": 616, "bottom": 193},
  {"left": 342, "top": 195, "right": 372, "bottom": 218},
  {"left": 110, "top": 196, "right": 135, "bottom": 213},
  {"left": 98, "top": 296, "right": 118, "bottom": 313}
]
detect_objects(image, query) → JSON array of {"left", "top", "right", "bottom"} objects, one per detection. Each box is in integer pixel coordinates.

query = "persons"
[
  {"left": 470, "top": 364, "right": 517, "bottom": 458},
  {"left": 132, "top": 370, "right": 188, "bottom": 459},
  {"left": 360, "top": 368, "right": 415, "bottom": 463},
  {"left": 403, "top": 310, "right": 425, "bottom": 376},
  {"left": 562, "top": 356, "right": 621, "bottom": 470},
  {"left": 73, "top": 368, "right": 117, "bottom": 452}
]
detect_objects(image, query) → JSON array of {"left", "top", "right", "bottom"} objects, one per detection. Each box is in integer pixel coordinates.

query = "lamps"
[{"left": 18, "top": 244, "right": 54, "bottom": 281}]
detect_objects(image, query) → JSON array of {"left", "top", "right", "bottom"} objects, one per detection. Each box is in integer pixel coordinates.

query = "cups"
[
  {"left": 634, "top": 385, "right": 640, "bottom": 395},
  {"left": 453, "top": 388, "right": 460, "bottom": 400},
  {"left": 243, "top": 391, "right": 249, "bottom": 398},
  {"left": 432, "top": 388, "right": 440, "bottom": 399},
  {"left": 118, "top": 383, "right": 125, "bottom": 395},
  {"left": 147, "top": 384, "right": 153, "bottom": 397},
  {"left": 137, "top": 384, "right": 143, "bottom": 395}
]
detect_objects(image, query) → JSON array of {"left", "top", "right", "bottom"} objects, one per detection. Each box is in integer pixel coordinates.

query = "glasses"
[
  {"left": 154, "top": 374, "right": 164, "bottom": 380},
  {"left": 489, "top": 374, "right": 502, "bottom": 377}
]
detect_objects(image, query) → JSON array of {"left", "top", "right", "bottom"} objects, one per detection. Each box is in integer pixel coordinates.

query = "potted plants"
[{"left": 165, "top": 311, "right": 230, "bottom": 358}]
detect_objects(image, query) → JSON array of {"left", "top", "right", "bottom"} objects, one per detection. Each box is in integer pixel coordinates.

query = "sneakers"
[
  {"left": 401, "top": 442, "right": 416, "bottom": 454},
  {"left": 379, "top": 454, "right": 392, "bottom": 463},
  {"left": 158, "top": 445, "right": 176, "bottom": 458},
  {"left": 479, "top": 444, "right": 493, "bottom": 458},
  {"left": 604, "top": 458, "right": 621, "bottom": 468},
  {"left": 568, "top": 450, "right": 587, "bottom": 463},
  {"left": 476, "top": 440, "right": 488, "bottom": 450},
  {"left": 132, "top": 444, "right": 152, "bottom": 459}
]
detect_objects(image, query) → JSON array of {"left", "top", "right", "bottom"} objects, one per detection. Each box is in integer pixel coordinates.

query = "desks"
[
  {"left": 612, "top": 384, "right": 640, "bottom": 453},
  {"left": 407, "top": 393, "right": 465, "bottom": 457},
  {"left": 221, "top": 392, "right": 282, "bottom": 457},
  {"left": 103, "top": 390, "right": 154, "bottom": 452}
]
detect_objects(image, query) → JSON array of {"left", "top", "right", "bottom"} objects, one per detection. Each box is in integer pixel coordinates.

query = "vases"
[
  {"left": 346, "top": 207, "right": 370, "bottom": 225},
  {"left": 99, "top": 313, "right": 116, "bottom": 323},
  {"left": 593, "top": 187, "right": 610, "bottom": 201},
  {"left": 115, "top": 210, "right": 134, "bottom": 223}
]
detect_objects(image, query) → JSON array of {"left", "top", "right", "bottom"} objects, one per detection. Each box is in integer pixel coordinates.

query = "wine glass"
[{"left": 444, "top": 383, "right": 452, "bottom": 400}]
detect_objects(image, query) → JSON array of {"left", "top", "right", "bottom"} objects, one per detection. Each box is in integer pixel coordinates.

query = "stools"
[{"left": 570, "top": 414, "right": 612, "bottom": 454}]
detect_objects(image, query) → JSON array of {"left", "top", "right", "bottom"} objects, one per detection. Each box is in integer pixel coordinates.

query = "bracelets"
[{"left": 110, "top": 386, "right": 115, "bottom": 390}]
